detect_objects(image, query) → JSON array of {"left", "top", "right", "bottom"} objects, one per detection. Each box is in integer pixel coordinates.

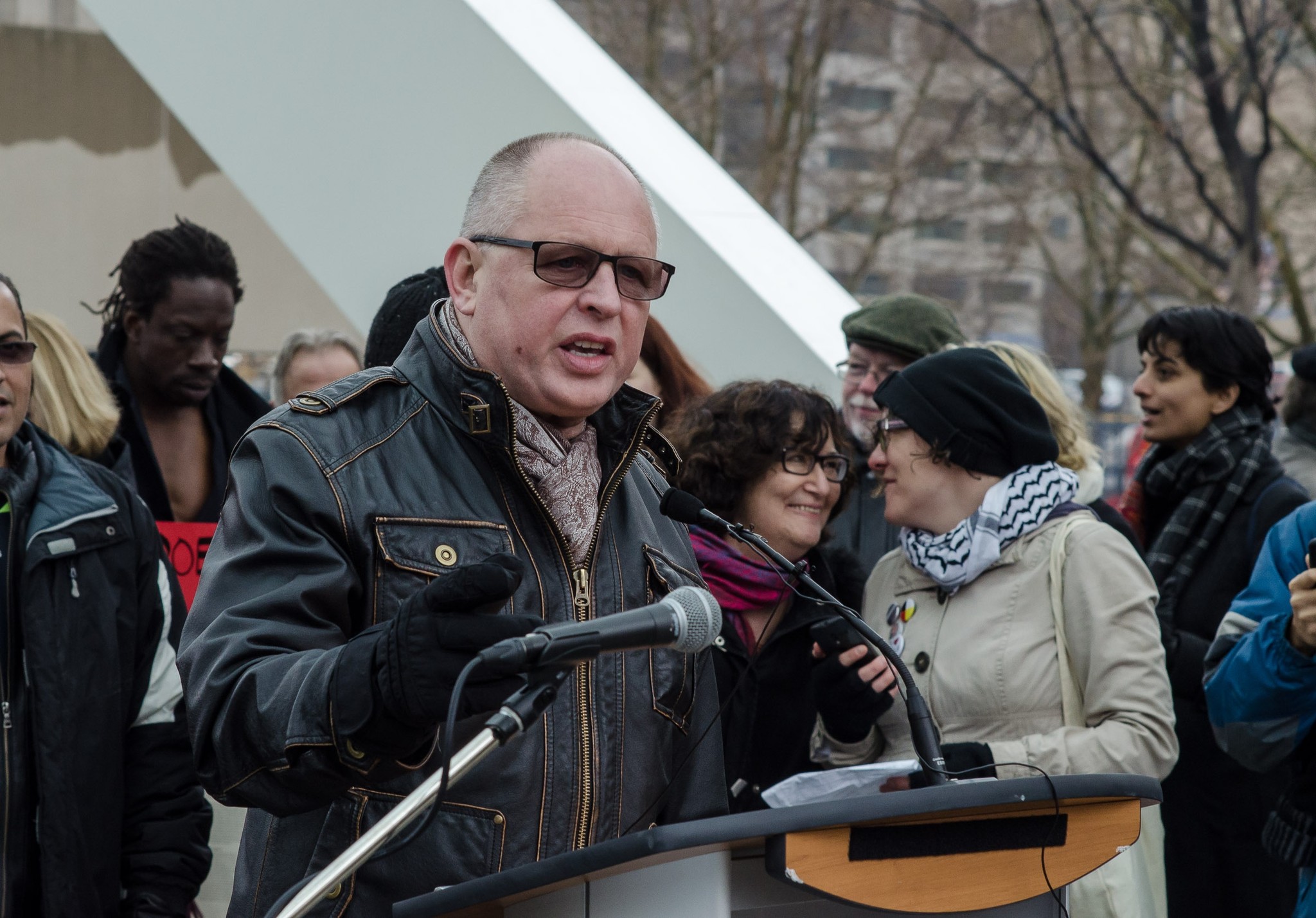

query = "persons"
[
  {"left": 176, "top": 133, "right": 730, "bottom": 918},
  {"left": 805, "top": 348, "right": 1180, "bottom": 918},
  {"left": 821, "top": 293, "right": 966, "bottom": 574},
  {"left": 87, "top": 221, "right": 274, "bottom": 616},
  {"left": 624, "top": 314, "right": 710, "bottom": 431},
  {"left": 271, "top": 330, "right": 363, "bottom": 404},
  {"left": 22, "top": 312, "right": 137, "bottom": 493},
  {"left": 367, "top": 264, "right": 452, "bottom": 367},
  {"left": 0, "top": 275, "right": 213, "bottom": 918},
  {"left": 1113, "top": 304, "right": 1316, "bottom": 918},
  {"left": 971, "top": 341, "right": 1143, "bottom": 558},
  {"left": 652, "top": 379, "right": 869, "bottom": 811}
]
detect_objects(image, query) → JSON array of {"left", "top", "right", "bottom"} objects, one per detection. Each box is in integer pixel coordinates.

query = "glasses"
[
  {"left": 836, "top": 360, "right": 898, "bottom": 382},
  {"left": 874, "top": 418, "right": 910, "bottom": 453},
  {"left": 776, "top": 446, "right": 851, "bottom": 483},
  {"left": 468, "top": 234, "right": 675, "bottom": 301},
  {"left": 0, "top": 342, "right": 38, "bottom": 364}
]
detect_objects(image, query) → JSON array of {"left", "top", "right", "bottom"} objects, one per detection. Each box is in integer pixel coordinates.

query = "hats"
[
  {"left": 871, "top": 347, "right": 1059, "bottom": 475},
  {"left": 841, "top": 294, "right": 969, "bottom": 359}
]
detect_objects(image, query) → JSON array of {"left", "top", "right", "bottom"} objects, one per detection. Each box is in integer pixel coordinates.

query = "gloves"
[{"left": 372, "top": 552, "right": 552, "bottom": 734}]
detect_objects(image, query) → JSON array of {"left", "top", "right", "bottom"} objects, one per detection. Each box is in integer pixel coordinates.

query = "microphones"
[
  {"left": 480, "top": 587, "right": 722, "bottom": 672},
  {"left": 659, "top": 488, "right": 950, "bottom": 783}
]
